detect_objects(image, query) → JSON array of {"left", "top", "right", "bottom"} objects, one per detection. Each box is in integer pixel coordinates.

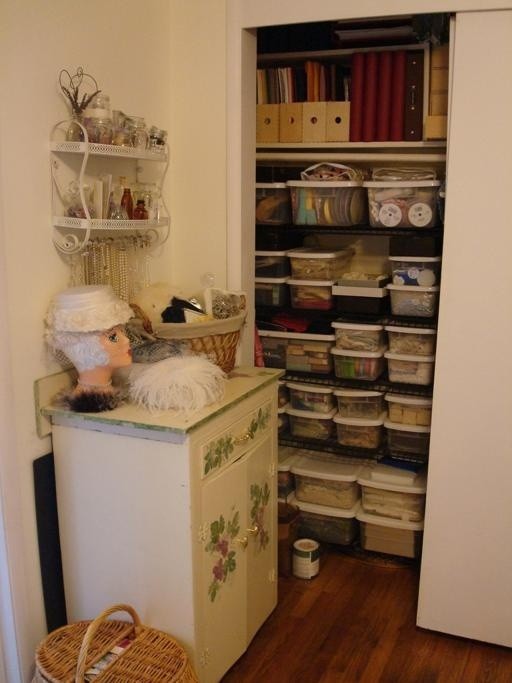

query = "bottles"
[
  {"left": 107, "top": 176, "right": 159, "bottom": 225},
  {"left": 293, "top": 539, "right": 321, "bottom": 581},
  {"left": 65, "top": 94, "right": 168, "bottom": 154}
]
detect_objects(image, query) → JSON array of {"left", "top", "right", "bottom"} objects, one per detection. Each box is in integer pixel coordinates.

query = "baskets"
[
  {"left": 32, "top": 604, "right": 197, "bottom": 683},
  {"left": 130, "top": 295, "right": 247, "bottom": 373}
]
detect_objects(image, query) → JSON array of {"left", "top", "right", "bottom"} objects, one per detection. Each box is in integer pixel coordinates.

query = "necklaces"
[
  {"left": 76, "top": 377, "right": 115, "bottom": 388},
  {"left": 81, "top": 238, "right": 152, "bottom": 301}
]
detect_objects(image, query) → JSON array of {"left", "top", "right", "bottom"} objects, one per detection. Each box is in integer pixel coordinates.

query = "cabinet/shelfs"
[
  {"left": 254, "top": 42, "right": 454, "bottom": 474},
  {"left": 41, "top": 365, "right": 287, "bottom": 683},
  {"left": 50, "top": 119, "right": 170, "bottom": 254}
]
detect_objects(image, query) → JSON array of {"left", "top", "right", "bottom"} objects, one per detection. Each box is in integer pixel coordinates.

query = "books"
[{"left": 255, "top": 49, "right": 423, "bottom": 142}]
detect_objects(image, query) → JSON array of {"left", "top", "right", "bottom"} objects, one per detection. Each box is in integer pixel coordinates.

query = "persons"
[{"left": 41, "top": 284, "right": 135, "bottom": 402}]
